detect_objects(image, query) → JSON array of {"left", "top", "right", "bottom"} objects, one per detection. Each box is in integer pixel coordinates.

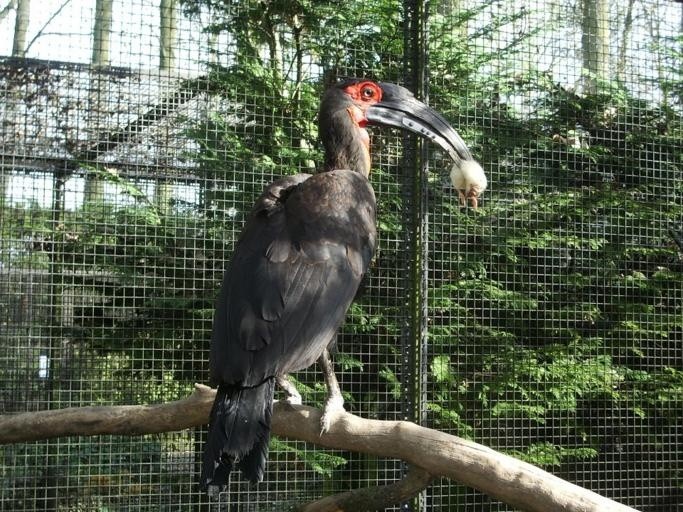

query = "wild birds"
[
  {"left": 449, "top": 159, "right": 488, "bottom": 210},
  {"left": 197, "top": 76, "right": 473, "bottom": 498}
]
